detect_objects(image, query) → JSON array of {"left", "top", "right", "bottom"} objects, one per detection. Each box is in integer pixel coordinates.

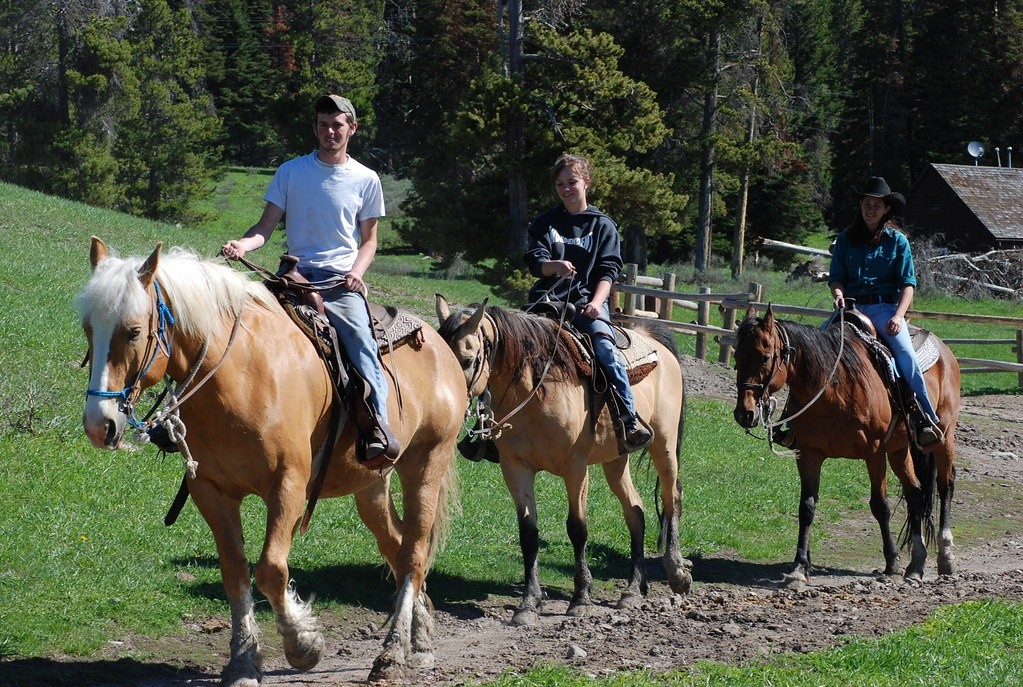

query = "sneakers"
[{"left": 617, "top": 427, "right": 651, "bottom": 455}]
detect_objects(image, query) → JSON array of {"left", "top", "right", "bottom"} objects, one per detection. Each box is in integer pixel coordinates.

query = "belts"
[{"left": 847, "top": 295, "right": 899, "bottom": 305}]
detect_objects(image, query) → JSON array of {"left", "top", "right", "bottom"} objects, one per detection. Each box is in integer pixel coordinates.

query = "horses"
[
  {"left": 733, "top": 302, "right": 961, "bottom": 590},
  {"left": 74, "top": 233, "right": 468, "bottom": 687},
  {"left": 436, "top": 293, "right": 686, "bottom": 628}
]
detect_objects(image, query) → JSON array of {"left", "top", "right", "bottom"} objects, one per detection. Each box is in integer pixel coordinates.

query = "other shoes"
[
  {"left": 366, "top": 427, "right": 395, "bottom": 469},
  {"left": 919, "top": 426, "right": 937, "bottom": 446}
]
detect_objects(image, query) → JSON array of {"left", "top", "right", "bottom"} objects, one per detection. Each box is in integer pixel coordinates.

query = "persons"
[
  {"left": 817, "top": 176, "right": 940, "bottom": 447},
  {"left": 521, "top": 153, "right": 651, "bottom": 456},
  {"left": 219, "top": 92, "right": 400, "bottom": 464}
]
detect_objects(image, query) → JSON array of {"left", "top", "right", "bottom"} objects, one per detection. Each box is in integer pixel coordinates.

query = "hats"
[
  {"left": 315, "top": 94, "right": 356, "bottom": 122},
  {"left": 850, "top": 176, "right": 906, "bottom": 212}
]
